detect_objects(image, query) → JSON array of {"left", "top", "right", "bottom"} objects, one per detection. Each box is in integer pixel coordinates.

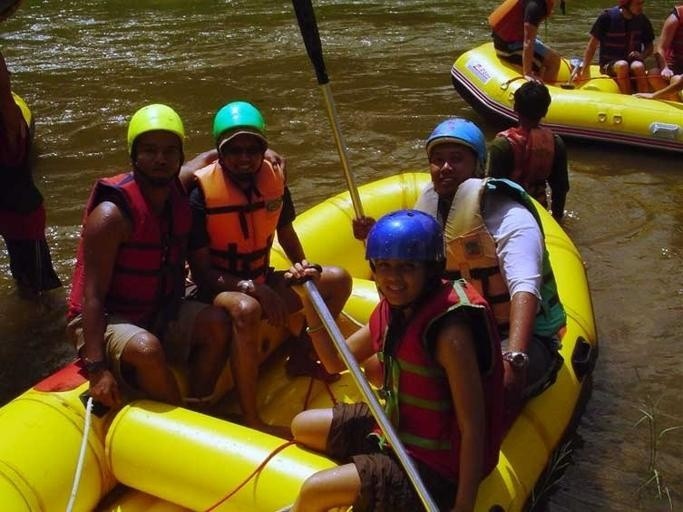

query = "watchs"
[
  {"left": 85, "top": 358, "right": 106, "bottom": 373},
  {"left": 503, "top": 351, "right": 529, "bottom": 370},
  {"left": 241, "top": 280, "right": 253, "bottom": 294}
]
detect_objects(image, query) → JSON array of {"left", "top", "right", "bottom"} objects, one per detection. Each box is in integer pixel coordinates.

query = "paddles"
[
  {"left": 291, "top": 0, "right": 371, "bottom": 248},
  {"left": 562, "top": 59, "right": 583, "bottom": 88}
]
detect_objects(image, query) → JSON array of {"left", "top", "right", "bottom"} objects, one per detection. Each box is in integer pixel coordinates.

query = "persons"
[
  {"left": 489, "top": 0, "right": 566, "bottom": 85},
  {"left": 653, "top": 3, "right": 683, "bottom": 80},
  {"left": 352, "top": 121, "right": 566, "bottom": 402},
  {"left": 572, "top": 0, "right": 656, "bottom": 94},
  {"left": 0, "top": 54, "right": 61, "bottom": 303},
  {"left": 67, "top": 104, "right": 286, "bottom": 443},
  {"left": 487, "top": 81, "right": 569, "bottom": 219},
  {"left": 277, "top": 211, "right": 503, "bottom": 512},
  {"left": 188, "top": 101, "right": 352, "bottom": 441},
  {"left": 635, "top": 75, "right": 683, "bottom": 104}
]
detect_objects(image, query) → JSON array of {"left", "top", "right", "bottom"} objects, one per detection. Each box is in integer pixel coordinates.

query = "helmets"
[
  {"left": 214, "top": 101, "right": 267, "bottom": 143},
  {"left": 126, "top": 103, "right": 187, "bottom": 160},
  {"left": 364, "top": 210, "right": 443, "bottom": 264},
  {"left": 10, "top": 90, "right": 35, "bottom": 137},
  {"left": 427, "top": 117, "right": 486, "bottom": 164}
]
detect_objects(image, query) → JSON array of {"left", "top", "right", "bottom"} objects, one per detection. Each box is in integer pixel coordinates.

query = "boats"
[
  {"left": 0, "top": 171, "right": 599, "bottom": 512},
  {"left": 450, "top": 41, "right": 683, "bottom": 154}
]
